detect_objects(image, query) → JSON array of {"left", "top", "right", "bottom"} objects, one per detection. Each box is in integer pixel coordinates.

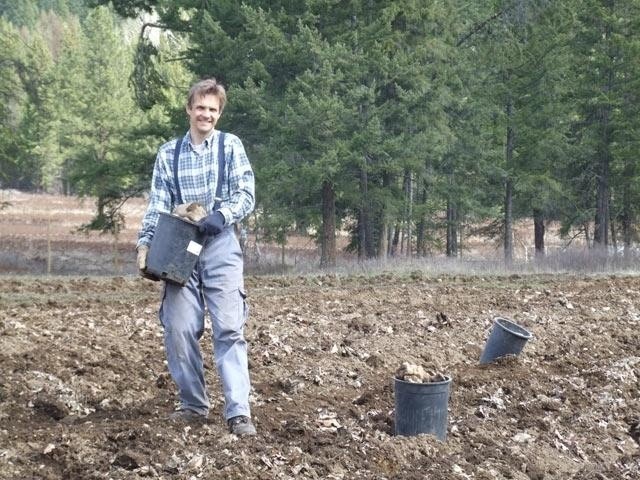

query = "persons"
[{"left": 137, "top": 79, "right": 258, "bottom": 436}]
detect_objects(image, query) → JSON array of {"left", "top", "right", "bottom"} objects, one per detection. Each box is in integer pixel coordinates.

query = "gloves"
[
  {"left": 195, "top": 211, "right": 227, "bottom": 237},
  {"left": 136, "top": 244, "right": 160, "bottom": 281}
]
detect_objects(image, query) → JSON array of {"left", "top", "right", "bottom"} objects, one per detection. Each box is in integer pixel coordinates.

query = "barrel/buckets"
[
  {"left": 394, "top": 376, "right": 452, "bottom": 443},
  {"left": 142, "top": 211, "right": 208, "bottom": 286},
  {"left": 480, "top": 317, "right": 531, "bottom": 367}
]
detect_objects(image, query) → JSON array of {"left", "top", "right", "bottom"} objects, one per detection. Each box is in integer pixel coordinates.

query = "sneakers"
[
  {"left": 167, "top": 408, "right": 209, "bottom": 423},
  {"left": 227, "top": 414, "right": 258, "bottom": 438}
]
